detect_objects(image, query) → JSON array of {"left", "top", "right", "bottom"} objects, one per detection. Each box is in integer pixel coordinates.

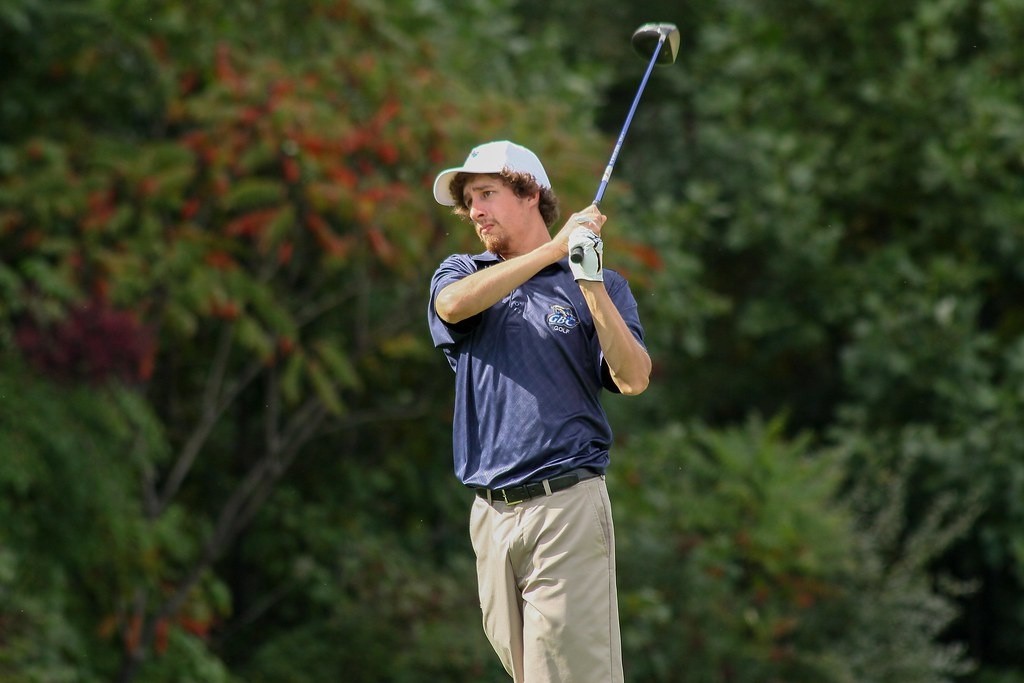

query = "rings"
[{"left": 576, "top": 216, "right": 587, "bottom": 224}]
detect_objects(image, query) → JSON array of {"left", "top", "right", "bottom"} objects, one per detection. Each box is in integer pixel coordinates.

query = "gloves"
[{"left": 567, "top": 225, "right": 603, "bottom": 282}]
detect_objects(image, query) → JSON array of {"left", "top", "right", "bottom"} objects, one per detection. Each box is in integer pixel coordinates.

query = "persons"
[{"left": 426, "top": 140, "right": 651, "bottom": 683}]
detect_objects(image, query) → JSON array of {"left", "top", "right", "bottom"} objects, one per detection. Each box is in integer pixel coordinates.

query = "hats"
[{"left": 433, "top": 141, "right": 552, "bottom": 207}]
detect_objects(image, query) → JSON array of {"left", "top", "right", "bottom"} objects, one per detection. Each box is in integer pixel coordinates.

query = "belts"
[{"left": 475, "top": 467, "right": 600, "bottom": 508}]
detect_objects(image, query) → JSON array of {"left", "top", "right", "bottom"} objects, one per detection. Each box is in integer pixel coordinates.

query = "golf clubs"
[{"left": 570, "top": 22, "right": 681, "bottom": 264}]
